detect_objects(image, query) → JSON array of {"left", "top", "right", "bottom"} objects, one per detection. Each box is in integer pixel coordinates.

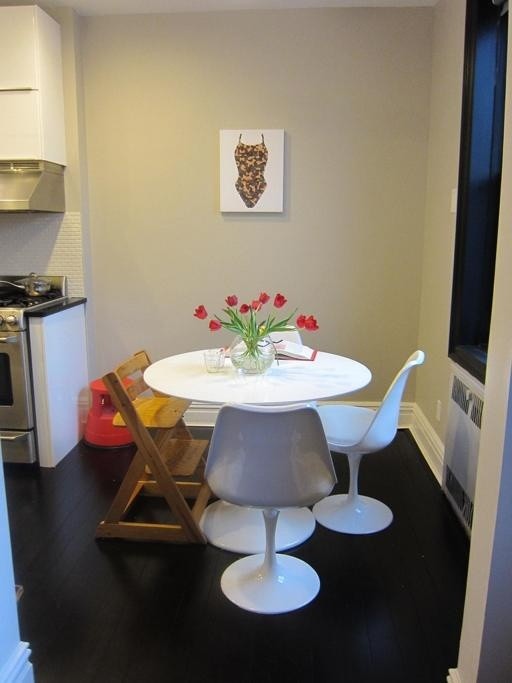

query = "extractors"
[{"left": 0, "top": 159, "right": 67, "bottom": 215}]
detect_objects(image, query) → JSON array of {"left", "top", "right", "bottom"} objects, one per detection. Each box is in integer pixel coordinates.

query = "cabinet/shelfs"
[{"left": 0, "top": 4, "right": 68, "bottom": 166}]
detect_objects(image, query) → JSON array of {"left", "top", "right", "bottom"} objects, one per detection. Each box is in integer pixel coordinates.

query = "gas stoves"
[{"left": 0, "top": 289, "right": 60, "bottom": 332}]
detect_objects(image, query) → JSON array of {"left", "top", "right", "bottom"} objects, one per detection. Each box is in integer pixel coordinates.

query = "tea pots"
[{"left": 0, "top": 272, "right": 52, "bottom": 296}]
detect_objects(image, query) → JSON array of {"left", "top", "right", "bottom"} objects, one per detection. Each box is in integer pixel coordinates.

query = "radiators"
[{"left": 439, "top": 362, "right": 484, "bottom": 540}]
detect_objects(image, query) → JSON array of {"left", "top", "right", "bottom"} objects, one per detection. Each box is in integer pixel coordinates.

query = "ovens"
[{"left": 0, "top": 332, "right": 37, "bottom": 465}]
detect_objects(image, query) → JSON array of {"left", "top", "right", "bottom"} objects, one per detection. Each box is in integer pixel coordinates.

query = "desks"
[{"left": 144, "top": 344, "right": 371, "bottom": 552}]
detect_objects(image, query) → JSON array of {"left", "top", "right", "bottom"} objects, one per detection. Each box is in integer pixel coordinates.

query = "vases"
[{"left": 230, "top": 332, "right": 275, "bottom": 374}]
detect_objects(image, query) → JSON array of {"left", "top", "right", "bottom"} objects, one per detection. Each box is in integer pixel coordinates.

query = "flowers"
[{"left": 193, "top": 289, "right": 320, "bottom": 363}]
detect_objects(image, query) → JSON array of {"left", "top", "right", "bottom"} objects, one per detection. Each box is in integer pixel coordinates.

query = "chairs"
[
  {"left": 201, "top": 401, "right": 337, "bottom": 614},
  {"left": 94, "top": 349, "right": 211, "bottom": 547},
  {"left": 309, "top": 348, "right": 427, "bottom": 535},
  {"left": 249, "top": 322, "right": 304, "bottom": 345}
]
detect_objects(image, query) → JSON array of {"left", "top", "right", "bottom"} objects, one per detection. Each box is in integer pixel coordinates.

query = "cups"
[
  {"left": 210, "top": 347, "right": 225, "bottom": 367},
  {"left": 202, "top": 351, "right": 220, "bottom": 373}
]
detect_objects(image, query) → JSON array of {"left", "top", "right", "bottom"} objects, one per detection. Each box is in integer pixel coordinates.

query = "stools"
[{"left": 84, "top": 376, "right": 139, "bottom": 451}]
